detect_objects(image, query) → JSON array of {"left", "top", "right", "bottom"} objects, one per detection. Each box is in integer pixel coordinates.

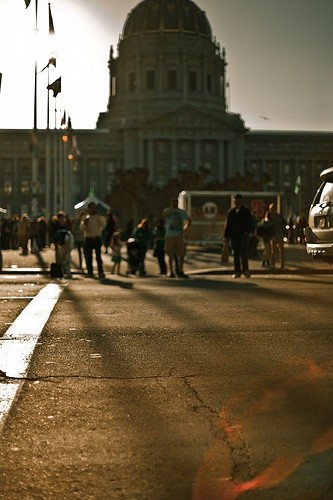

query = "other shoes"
[
  {"left": 244, "top": 273, "right": 250, "bottom": 279},
  {"left": 67, "top": 273, "right": 72, "bottom": 278},
  {"left": 233, "top": 272, "right": 242, "bottom": 279},
  {"left": 99, "top": 273, "right": 105, "bottom": 278},
  {"left": 177, "top": 272, "right": 188, "bottom": 278},
  {"left": 169, "top": 273, "right": 175, "bottom": 278},
  {"left": 88, "top": 271, "right": 95, "bottom": 278}
]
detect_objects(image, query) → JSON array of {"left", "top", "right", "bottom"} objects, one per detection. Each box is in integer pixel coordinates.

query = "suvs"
[{"left": 303, "top": 167, "right": 333, "bottom": 259}]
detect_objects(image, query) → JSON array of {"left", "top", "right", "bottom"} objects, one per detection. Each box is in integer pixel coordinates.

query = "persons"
[
  {"left": 79, "top": 202, "right": 111, "bottom": 284},
  {"left": 223, "top": 193, "right": 258, "bottom": 280},
  {"left": 261, "top": 200, "right": 308, "bottom": 268},
  {"left": 162, "top": 195, "right": 192, "bottom": 278},
  {"left": 0, "top": 210, "right": 169, "bottom": 278}
]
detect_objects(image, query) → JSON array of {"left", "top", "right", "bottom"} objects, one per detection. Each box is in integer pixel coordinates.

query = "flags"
[{"left": 23, "top": 0, "right": 75, "bottom": 138}]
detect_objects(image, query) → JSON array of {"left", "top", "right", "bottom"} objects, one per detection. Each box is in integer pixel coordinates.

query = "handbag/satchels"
[
  {"left": 248, "top": 228, "right": 258, "bottom": 258},
  {"left": 51, "top": 262, "right": 63, "bottom": 278},
  {"left": 220, "top": 238, "right": 229, "bottom": 262}
]
